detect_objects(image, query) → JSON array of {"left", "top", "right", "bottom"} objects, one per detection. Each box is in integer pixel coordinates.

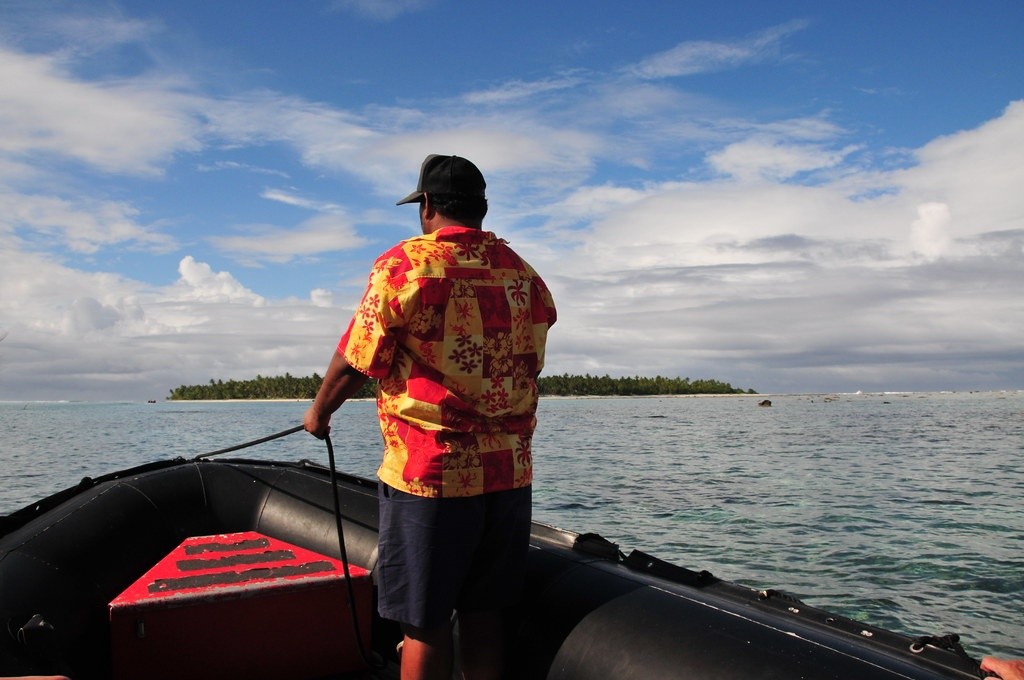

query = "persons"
[{"left": 303, "top": 154, "right": 558, "bottom": 680}]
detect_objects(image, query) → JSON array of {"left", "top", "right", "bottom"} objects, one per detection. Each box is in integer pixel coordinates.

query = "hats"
[{"left": 396, "top": 154, "right": 487, "bottom": 208}]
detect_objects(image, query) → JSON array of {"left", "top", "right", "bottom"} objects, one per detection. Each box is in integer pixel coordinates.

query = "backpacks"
[{"left": 0, "top": 612, "right": 78, "bottom": 680}]
